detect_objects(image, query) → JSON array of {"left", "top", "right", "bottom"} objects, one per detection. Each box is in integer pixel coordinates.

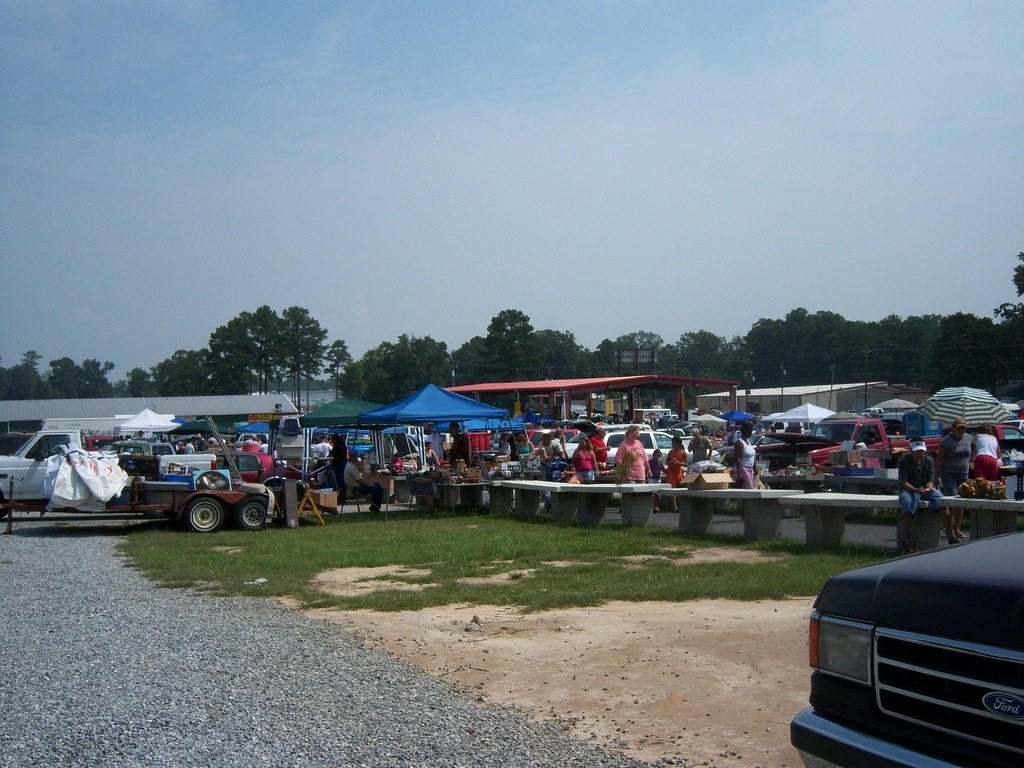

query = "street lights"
[
  {"left": 828, "top": 362, "right": 838, "bottom": 411},
  {"left": 861, "top": 344, "right": 872, "bottom": 409}
]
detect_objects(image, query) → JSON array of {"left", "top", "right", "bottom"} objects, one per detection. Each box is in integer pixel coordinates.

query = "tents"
[
  {"left": 720, "top": 410, "right": 757, "bottom": 425},
  {"left": 760, "top": 404, "right": 837, "bottom": 434},
  {"left": 300, "top": 384, "right": 560, "bottom": 452},
  {"left": 121, "top": 409, "right": 282, "bottom": 442},
  {"left": 690, "top": 414, "right": 728, "bottom": 425}
]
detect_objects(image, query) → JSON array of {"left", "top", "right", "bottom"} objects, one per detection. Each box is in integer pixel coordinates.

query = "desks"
[
  {"left": 500, "top": 475, "right": 1024, "bottom": 554},
  {"left": 432, "top": 483, "right": 491, "bottom": 517},
  {"left": 362, "top": 472, "right": 406, "bottom": 519}
]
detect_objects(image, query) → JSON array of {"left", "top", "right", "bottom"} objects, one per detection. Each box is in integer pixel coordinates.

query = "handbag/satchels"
[{"left": 752, "top": 475, "right": 765, "bottom": 489}]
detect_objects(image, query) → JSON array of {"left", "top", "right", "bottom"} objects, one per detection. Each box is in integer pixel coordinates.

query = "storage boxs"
[
  {"left": 158, "top": 460, "right": 196, "bottom": 490},
  {"left": 309, "top": 490, "right": 338, "bottom": 506},
  {"left": 469, "top": 432, "right": 490, "bottom": 451},
  {"left": 441, "top": 458, "right": 476, "bottom": 478},
  {"left": 832, "top": 450, "right": 886, "bottom": 476},
  {"left": 680, "top": 470, "right": 735, "bottom": 490}
]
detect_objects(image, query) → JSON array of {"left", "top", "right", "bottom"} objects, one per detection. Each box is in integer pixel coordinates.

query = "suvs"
[{"left": 603, "top": 430, "right": 721, "bottom": 472}]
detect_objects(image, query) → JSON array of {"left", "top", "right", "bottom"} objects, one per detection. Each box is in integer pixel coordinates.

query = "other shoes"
[{"left": 955, "top": 531, "right": 968, "bottom": 538}]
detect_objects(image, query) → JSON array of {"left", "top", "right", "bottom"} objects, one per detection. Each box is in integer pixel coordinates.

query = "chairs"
[{"left": 345, "top": 483, "right": 381, "bottom": 513}]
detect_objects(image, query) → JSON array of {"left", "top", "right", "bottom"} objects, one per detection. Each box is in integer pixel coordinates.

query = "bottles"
[
  {"left": 88, "top": 425, "right": 121, "bottom": 436},
  {"left": 94, "top": 440, "right": 99, "bottom": 448},
  {"left": 162, "top": 463, "right": 189, "bottom": 475},
  {"left": 80, "top": 430, "right": 87, "bottom": 446},
  {"left": 313, "top": 450, "right": 319, "bottom": 459}
]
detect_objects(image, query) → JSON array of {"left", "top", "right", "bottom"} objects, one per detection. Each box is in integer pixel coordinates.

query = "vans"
[{"left": 861, "top": 407, "right": 884, "bottom": 418}]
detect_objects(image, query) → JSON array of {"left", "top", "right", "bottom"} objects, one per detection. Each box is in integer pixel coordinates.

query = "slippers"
[
  {"left": 943, "top": 527, "right": 960, "bottom": 545},
  {"left": 898, "top": 537, "right": 918, "bottom": 554}
]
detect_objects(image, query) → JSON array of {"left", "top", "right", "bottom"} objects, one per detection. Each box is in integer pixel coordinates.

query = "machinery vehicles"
[{"left": 0, "top": 476, "right": 271, "bottom": 532}]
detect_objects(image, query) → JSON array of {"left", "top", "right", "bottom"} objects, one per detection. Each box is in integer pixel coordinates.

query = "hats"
[
  {"left": 540, "top": 434, "right": 554, "bottom": 440},
  {"left": 909, "top": 442, "right": 926, "bottom": 451},
  {"left": 954, "top": 418, "right": 967, "bottom": 428}
]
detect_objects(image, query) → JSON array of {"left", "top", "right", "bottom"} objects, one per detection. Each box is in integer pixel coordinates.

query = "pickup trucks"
[
  {"left": 0, "top": 429, "right": 217, "bottom": 518},
  {"left": 842, "top": 410, "right": 870, "bottom": 418},
  {"left": 211, "top": 451, "right": 320, "bottom": 490},
  {"left": 753, "top": 419, "right": 942, "bottom": 471}
]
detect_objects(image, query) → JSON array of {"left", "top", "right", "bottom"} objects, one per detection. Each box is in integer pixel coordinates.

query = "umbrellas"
[
  {"left": 871, "top": 399, "right": 919, "bottom": 419},
  {"left": 915, "top": 387, "right": 1014, "bottom": 424}
]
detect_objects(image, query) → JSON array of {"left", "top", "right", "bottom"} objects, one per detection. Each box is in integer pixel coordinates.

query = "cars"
[
  {"left": 790, "top": 530, "right": 1024, "bottom": 768},
  {"left": 568, "top": 406, "right": 699, "bottom": 438},
  {"left": 527, "top": 429, "right": 581, "bottom": 451},
  {"left": 84, "top": 434, "right": 269, "bottom": 456},
  {"left": 716, "top": 436, "right": 787, "bottom": 466},
  {"left": 983, "top": 379, "right": 1024, "bottom": 419},
  {"left": 560, "top": 420, "right": 652, "bottom": 464},
  {"left": 751, "top": 416, "right": 825, "bottom": 438},
  {"left": 993, "top": 425, "right": 1024, "bottom": 440},
  {"left": 1000, "top": 420, "right": 1024, "bottom": 431},
  {"left": 0, "top": 436, "right": 32, "bottom": 456}
]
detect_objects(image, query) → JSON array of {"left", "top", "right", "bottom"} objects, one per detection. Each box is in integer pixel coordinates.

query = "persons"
[
  {"left": 425, "top": 441, "right": 441, "bottom": 466},
  {"left": 344, "top": 451, "right": 383, "bottom": 513},
  {"left": 118, "top": 431, "right": 269, "bottom": 455},
  {"left": 897, "top": 419, "right": 1001, "bottom": 554},
  {"left": 310, "top": 432, "right": 347, "bottom": 504},
  {"left": 515, "top": 434, "right": 535, "bottom": 456},
  {"left": 539, "top": 427, "right": 611, "bottom": 483},
  {"left": 391, "top": 453, "right": 404, "bottom": 473},
  {"left": 732, "top": 421, "right": 759, "bottom": 489},
  {"left": 615, "top": 426, "right": 713, "bottom": 513},
  {"left": 495, "top": 433, "right": 511, "bottom": 456}
]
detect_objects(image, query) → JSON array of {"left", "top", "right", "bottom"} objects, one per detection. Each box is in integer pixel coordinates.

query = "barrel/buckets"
[{"left": 241, "top": 443, "right": 259, "bottom": 453}]
